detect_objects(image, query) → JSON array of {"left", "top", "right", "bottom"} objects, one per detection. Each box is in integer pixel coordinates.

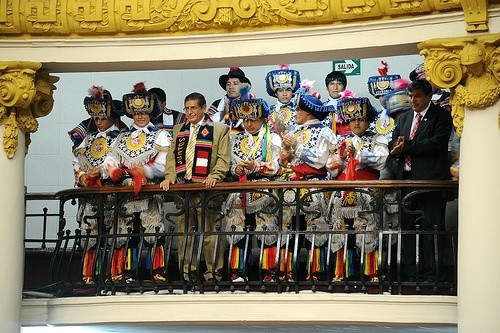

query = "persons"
[
  {"left": 263, "top": 63, "right": 305, "bottom": 135},
  {"left": 272, "top": 95, "right": 339, "bottom": 282},
  {"left": 204, "top": 66, "right": 260, "bottom": 181},
  {"left": 325, "top": 102, "right": 391, "bottom": 283},
  {"left": 388, "top": 77, "right": 454, "bottom": 281},
  {"left": 145, "top": 86, "right": 189, "bottom": 138},
  {"left": 71, "top": 100, "right": 136, "bottom": 286},
  {"left": 66, "top": 89, "right": 130, "bottom": 150},
  {"left": 317, "top": 69, "right": 353, "bottom": 116},
  {"left": 367, "top": 72, "right": 403, "bottom": 141},
  {"left": 158, "top": 92, "right": 232, "bottom": 282},
  {"left": 381, "top": 86, "right": 414, "bottom": 142},
  {"left": 224, "top": 103, "right": 289, "bottom": 283},
  {"left": 460, "top": 42, "right": 498, "bottom": 92},
  {"left": 102, "top": 92, "right": 173, "bottom": 281},
  {"left": 409, "top": 63, "right": 451, "bottom": 119}
]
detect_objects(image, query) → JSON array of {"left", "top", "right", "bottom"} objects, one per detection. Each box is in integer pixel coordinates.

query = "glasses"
[{"left": 183, "top": 105, "right": 200, "bottom": 112}]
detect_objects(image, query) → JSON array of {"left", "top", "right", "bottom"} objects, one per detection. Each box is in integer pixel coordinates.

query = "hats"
[
  {"left": 218, "top": 67, "right": 252, "bottom": 92},
  {"left": 294, "top": 88, "right": 335, "bottom": 120},
  {"left": 336, "top": 90, "right": 379, "bottom": 123},
  {"left": 409, "top": 63, "right": 430, "bottom": 81},
  {"left": 84, "top": 86, "right": 123, "bottom": 118},
  {"left": 229, "top": 87, "right": 269, "bottom": 120},
  {"left": 266, "top": 64, "right": 300, "bottom": 97},
  {"left": 382, "top": 78, "right": 414, "bottom": 115},
  {"left": 123, "top": 83, "right": 161, "bottom": 119},
  {"left": 368, "top": 61, "right": 401, "bottom": 99}
]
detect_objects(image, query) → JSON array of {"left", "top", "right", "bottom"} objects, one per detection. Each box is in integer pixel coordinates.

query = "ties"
[
  {"left": 184, "top": 125, "right": 199, "bottom": 180},
  {"left": 404, "top": 112, "right": 422, "bottom": 167}
]
